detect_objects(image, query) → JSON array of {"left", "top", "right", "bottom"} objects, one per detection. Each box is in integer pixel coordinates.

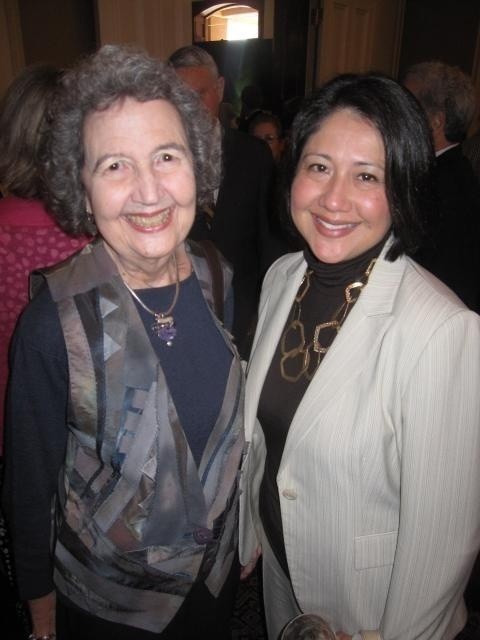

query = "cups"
[{"left": 281, "top": 613, "right": 337, "bottom": 639}]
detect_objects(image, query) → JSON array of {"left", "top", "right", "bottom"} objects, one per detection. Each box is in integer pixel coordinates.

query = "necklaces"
[
  {"left": 123, "top": 254, "right": 179, "bottom": 347},
  {"left": 281, "top": 259, "right": 379, "bottom": 383}
]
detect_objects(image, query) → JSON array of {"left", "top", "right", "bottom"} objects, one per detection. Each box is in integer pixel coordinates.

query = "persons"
[
  {"left": 401, "top": 62, "right": 479, "bottom": 311},
  {"left": 0, "top": 67, "right": 88, "bottom": 389},
  {"left": 171, "top": 46, "right": 306, "bottom": 358},
  {"left": 237, "top": 69, "right": 480, "bottom": 639},
  {"left": 0, "top": 44, "right": 246, "bottom": 640}
]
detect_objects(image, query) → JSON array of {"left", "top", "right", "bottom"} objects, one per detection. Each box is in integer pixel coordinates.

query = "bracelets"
[{"left": 28, "top": 633, "right": 58, "bottom": 640}]
